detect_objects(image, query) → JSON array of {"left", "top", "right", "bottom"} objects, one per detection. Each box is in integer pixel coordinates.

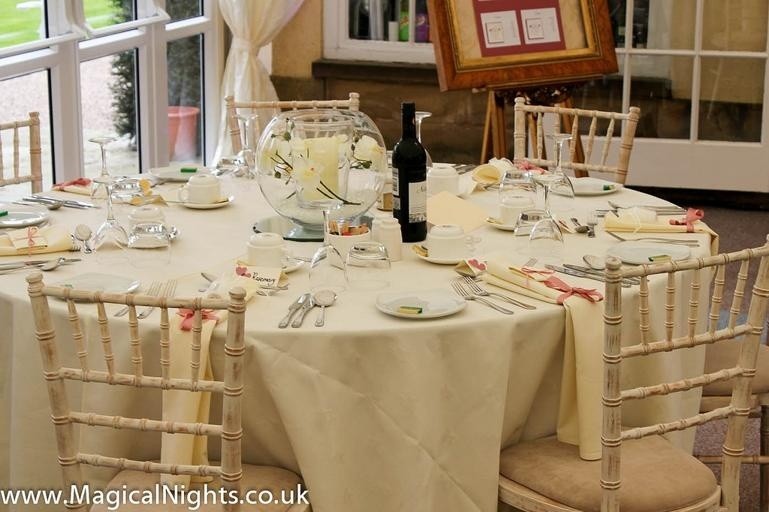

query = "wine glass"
[
  {"left": 412, "top": 110, "right": 434, "bottom": 167},
  {"left": 88, "top": 136, "right": 118, "bottom": 201},
  {"left": 544, "top": 129, "right": 577, "bottom": 216},
  {"left": 307, "top": 197, "right": 351, "bottom": 296},
  {"left": 232, "top": 111, "right": 260, "bottom": 178}
]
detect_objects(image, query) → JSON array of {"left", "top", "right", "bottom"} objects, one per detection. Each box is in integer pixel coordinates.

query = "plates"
[
  {"left": 178, "top": 174, "right": 221, "bottom": 204},
  {"left": 146, "top": 162, "right": 211, "bottom": 179},
  {"left": 370, "top": 289, "right": 469, "bottom": 322},
  {"left": 0, "top": 211, "right": 50, "bottom": 228},
  {"left": 603, "top": 245, "right": 693, "bottom": 265},
  {"left": 386, "top": 151, "right": 393, "bottom": 167},
  {"left": 184, "top": 196, "right": 236, "bottom": 210}
]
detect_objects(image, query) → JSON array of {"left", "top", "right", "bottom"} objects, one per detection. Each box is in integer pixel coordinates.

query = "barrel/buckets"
[{"left": 167, "top": 106, "right": 200, "bottom": 161}]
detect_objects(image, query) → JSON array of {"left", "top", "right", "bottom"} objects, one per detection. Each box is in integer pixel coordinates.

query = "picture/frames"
[{"left": 426, "top": 0, "right": 620, "bottom": 92}]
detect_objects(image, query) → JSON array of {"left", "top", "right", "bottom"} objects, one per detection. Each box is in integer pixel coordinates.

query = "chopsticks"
[
  {"left": 592, "top": 212, "right": 691, "bottom": 219},
  {"left": 12, "top": 194, "right": 102, "bottom": 211}
]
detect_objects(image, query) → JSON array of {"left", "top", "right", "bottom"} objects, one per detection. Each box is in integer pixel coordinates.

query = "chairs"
[
  {"left": 692, "top": 321, "right": 769, "bottom": 512},
  {"left": 0, "top": 111, "right": 43, "bottom": 193},
  {"left": 25, "top": 271, "right": 309, "bottom": 511},
  {"left": 513, "top": 95, "right": 641, "bottom": 183},
  {"left": 499, "top": 234, "right": 769, "bottom": 512},
  {"left": 225, "top": 92, "right": 359, "bottom": 155}
]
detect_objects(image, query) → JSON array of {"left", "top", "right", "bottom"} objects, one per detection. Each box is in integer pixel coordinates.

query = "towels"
[
  {"left": 483, "top": 263, "right": 606, "bottom": 462},
  {"left": 0, "top": 225, "right": 80, "bottom": 257},
  {"left": 605, "top": 208, "right": 719, "bottom": 280},
  {"left": 473, "top": 159, "right": 545, "bottom": 183},
  {"left": 159, "top": 273, "right": 259, "bottom": 490},
  {"left": 53, "top": 177, "right": 95, "bottom": 196}
]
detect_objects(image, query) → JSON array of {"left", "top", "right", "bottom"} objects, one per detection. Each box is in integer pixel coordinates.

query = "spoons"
[
  {"left": 585, "top": 255, "right": 612, "bottom": 269},
  {"left": 592, "top": 199, "right": 686, "bottom": 209},
  {"left": 200, "top": 273, "right": 287, "bottom": 294},
  {"left": 118, "top": 282, "right": 140, "bottom": 320},
  {"left": 313, "top": 287, "right": 336, "bottom": 326}
]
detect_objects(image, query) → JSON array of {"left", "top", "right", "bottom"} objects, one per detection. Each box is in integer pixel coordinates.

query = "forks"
[
  {"left": 604, "top": 230, "right": 705, "bottom": 242},
  {"left": 139, "top": 282, "right": 182, "bottom": 318},
  {"left": 462, "top": 274, "right": 537, "bottom": 311},
  {"left": 450, "top": 282, "right": 514, "bottom": 315}
]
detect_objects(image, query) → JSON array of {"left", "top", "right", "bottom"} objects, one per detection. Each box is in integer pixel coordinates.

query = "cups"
[
  {"left": 427, "top": 164, "right": 459, "bottom": 194},
  {"left": 231, "top": 171, "right": 480, "bottom": 278},
  {"left": 483, "top": 169, "right": 580, "bottom": 287},
  {"left": 90, "top": 175, "right": 181, "bottom": 281}
]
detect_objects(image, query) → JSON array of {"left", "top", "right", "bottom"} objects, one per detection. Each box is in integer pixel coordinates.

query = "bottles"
[{"left": 392, "top": 100, "right": 427, "bottom": 244}]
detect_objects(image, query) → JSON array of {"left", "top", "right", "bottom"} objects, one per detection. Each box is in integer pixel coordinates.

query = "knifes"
[
  {"left": 545, "top": 260, "right": 640, "bottom": 288},
  {"left": 293, "top": 297, "right": 316, "bottom": 327},
  {"left": 0, "top": 260, "right": 81, "bottom": 271},
  {"left": 278, "top": 292, "right": 312, "bottom": 328}
]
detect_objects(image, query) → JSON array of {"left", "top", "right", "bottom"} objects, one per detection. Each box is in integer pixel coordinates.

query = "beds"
[{"left": 0, "top": 162, "right": 722, "bottom": 512}]
